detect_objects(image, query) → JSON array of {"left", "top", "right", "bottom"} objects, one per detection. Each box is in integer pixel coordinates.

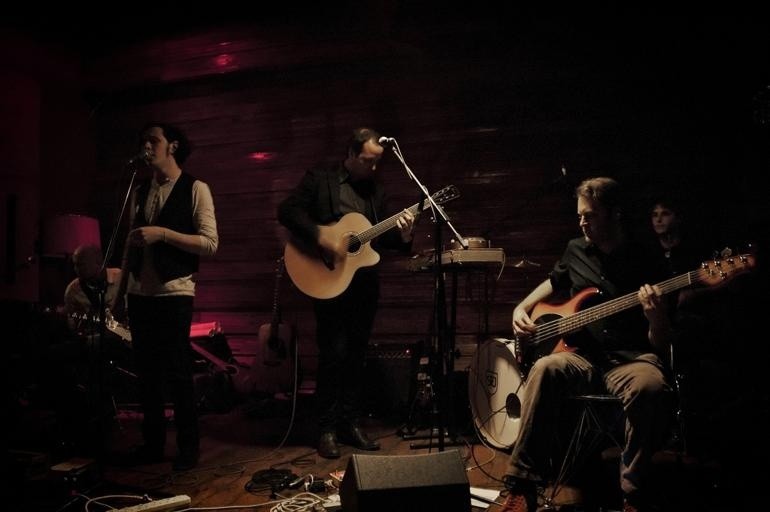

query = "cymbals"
[{"left": 507, "top": 257, "right": 540, "bottom": 269}]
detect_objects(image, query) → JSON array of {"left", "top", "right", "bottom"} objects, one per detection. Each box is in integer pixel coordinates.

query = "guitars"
[
  {"left": 283, "top": 185, "right": 460, "bottom": 301},
  {"left": 190, "top": 341, "right": 257, "bottom": 397},
  {"left": 515, "top": 247, "right": 756, "bottom": 371},
  {"left": 258, "top": 256, "right": 300, "bottom": 386}
]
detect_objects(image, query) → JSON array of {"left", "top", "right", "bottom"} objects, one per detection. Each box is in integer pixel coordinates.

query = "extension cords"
[{"left": 106, "top": 494, "right": 191, "bottom": 512}]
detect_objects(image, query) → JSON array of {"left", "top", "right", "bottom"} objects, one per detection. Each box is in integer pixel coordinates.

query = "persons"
[
  {"left": 110, "top": 124, "right": 219, "bottom": 471},
  {"left": 277, "top": 128, "right": 415, "bottom": 459},
  {"left": 651, "top": 203, "right": 691, "bottom": 258},
  {"left": 499, "top": 176, "right": 670, "bottom": 511},
  {"left": 64, "top": 243, "right": 122, "bottom": 314}
]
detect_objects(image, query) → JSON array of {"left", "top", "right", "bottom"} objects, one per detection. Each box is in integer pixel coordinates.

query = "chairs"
[{"left": 551, "top": 344, "right": 684, "bottom": 504}]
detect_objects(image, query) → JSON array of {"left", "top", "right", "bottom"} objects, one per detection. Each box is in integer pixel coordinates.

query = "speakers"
[
  {"left": 338, "top": 449, "right": 472, "bottom": 512},
  {"left": 361, "top": 341, "right": 422, "bottom": 416}
]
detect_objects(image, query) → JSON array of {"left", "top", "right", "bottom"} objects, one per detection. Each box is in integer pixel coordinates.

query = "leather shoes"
[
  {"left": 496, "top": 483, "right": 537, "bottom": 512},
  {"left": 318, "top": 427, "right": 380, "bottom": 457},
  {"left": 623, "top": 496, "right": 639, "bottom": 512},
  {"left": 126, "top": 441, "right": 200, "bottom": 471}
]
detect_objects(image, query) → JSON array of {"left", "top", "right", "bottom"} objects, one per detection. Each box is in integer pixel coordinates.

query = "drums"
[{"left": 468, "top": 335, "right": 529, "bottom": 453}]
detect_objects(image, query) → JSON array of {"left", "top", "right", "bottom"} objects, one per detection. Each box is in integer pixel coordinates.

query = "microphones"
[
  {"left": 129, "top": 152, "right": 150, "bottom": 163},
  {"left": 378, "top": 136, "right": 395, "bottom": 146}
]
